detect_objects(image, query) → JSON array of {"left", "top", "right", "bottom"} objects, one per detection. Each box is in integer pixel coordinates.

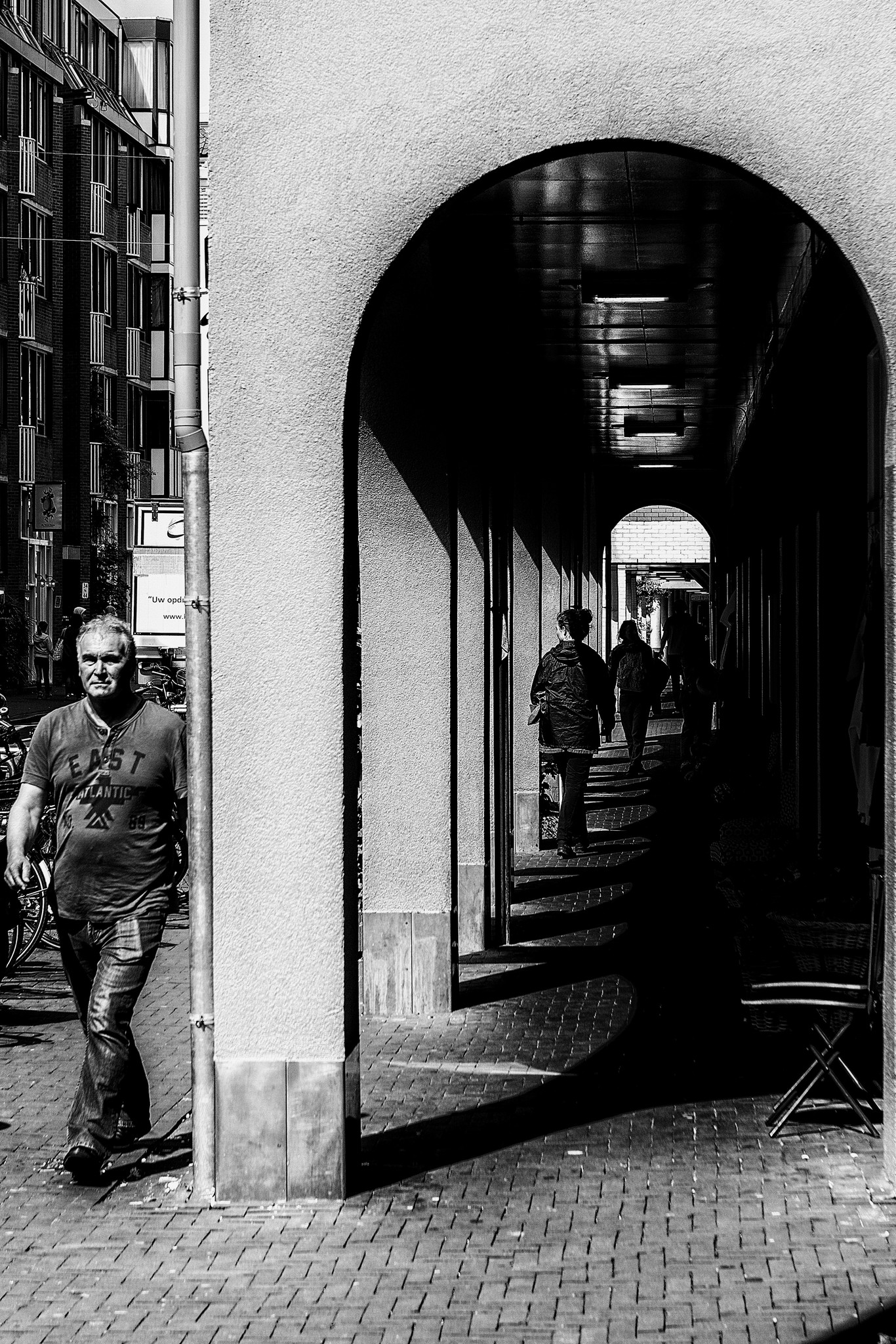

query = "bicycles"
[{"left": 0, "top": 649, "right": 189, "bottom": 990}]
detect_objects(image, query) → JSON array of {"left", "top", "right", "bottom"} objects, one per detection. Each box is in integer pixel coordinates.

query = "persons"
[
  {"left": 650, "top": 657, "right": 670, "bottom": 719},
  {"left": 68, "top": 607, "right": 87, "bottom": 628},
  {"left": 677, "top": 621, "right": 720, "bottom": 770},
  {"left": 32, "top": 621, "right": 55, "bottom": 700},
  {"left": 658, "top": 599, "right": 694, "bottom": 711},
  {"left": 105, "top": 606, "right": 116, "bottom": 617},
  {"left": 529, "top": 609, "right": 617, "bottom": 858},
  {"left": 4, "top": 609, "right": 187, "bottom": 1183},
  {"left": 606, "top": 620, "right": 656, "bottom": 780},
  {"left": 57, "top": 613, "right": 84, "bottom": 702}
]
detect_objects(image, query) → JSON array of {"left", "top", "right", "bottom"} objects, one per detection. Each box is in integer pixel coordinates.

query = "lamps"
[
  {"left": 34, "top": 572, "right": 45, "bottom": 588},
  {"left": 44, "top": 574, "right": 59, "bottom": 588}
]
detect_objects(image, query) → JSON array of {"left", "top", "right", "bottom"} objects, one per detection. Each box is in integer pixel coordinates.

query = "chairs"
[{"left": 735, "top": 855, "right": 884, "bottom": 1136}]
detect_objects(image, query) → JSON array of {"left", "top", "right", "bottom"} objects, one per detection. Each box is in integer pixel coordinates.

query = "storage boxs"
[{"left": 749, "top": 882, "right": 885, "bottom": 1035}]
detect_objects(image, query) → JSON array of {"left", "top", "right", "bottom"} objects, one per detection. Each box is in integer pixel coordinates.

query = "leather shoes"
[
  {"left": 114, "top": 1121, "right": 153, "bottom": 1150},
  {"left": 62, "top": 1146, "right": 102, "bottom": 1184}
]
